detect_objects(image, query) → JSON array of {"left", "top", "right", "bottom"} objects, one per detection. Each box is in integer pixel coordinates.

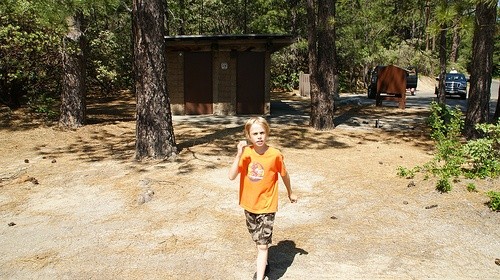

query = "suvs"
[
  {"left": 434, "top": 71, "right": 470, "bottom": 100},
  {"left": 368, "top": 66, "right": 417, "bottom": 100}
]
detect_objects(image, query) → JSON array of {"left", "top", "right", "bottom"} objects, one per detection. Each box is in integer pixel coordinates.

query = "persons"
[{"left": 228, "top": 114, "right": 299, "bottom": 280}]
